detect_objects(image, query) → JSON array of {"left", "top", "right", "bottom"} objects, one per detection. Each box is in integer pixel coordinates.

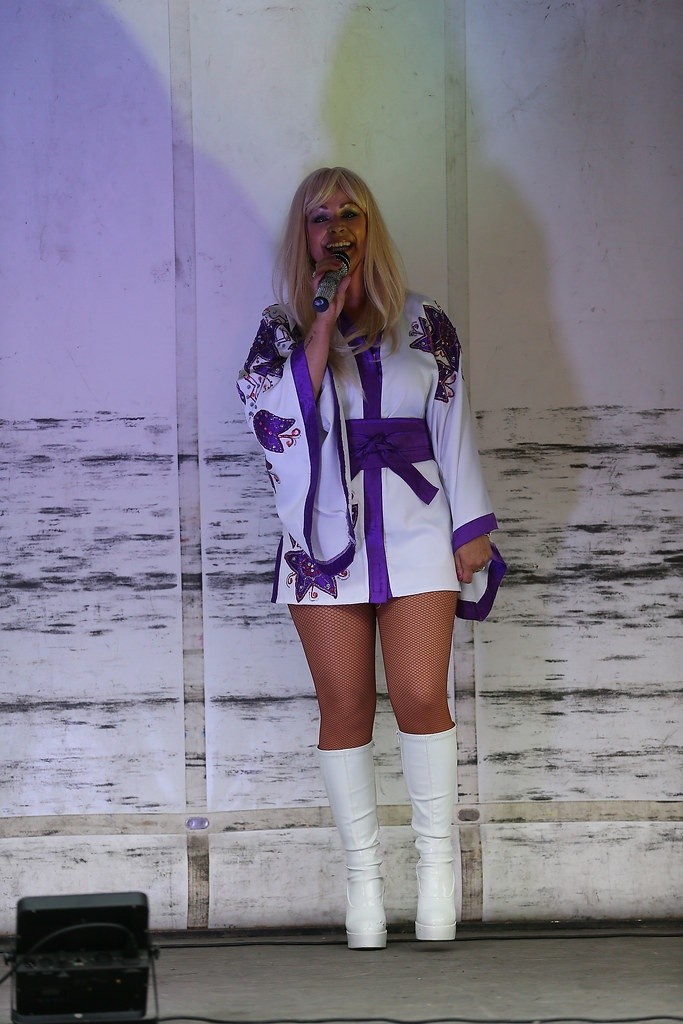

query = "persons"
[{"left": 237, "top": 166, "right": 495, "bottom": 953}]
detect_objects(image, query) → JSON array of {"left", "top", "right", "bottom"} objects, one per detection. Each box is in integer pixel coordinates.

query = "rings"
[
  {"left": 472, "top": 566, "right": 485, "bottom": 573},
  {"left": 311, "top": 263, "right": 318, "bottom": 278}
]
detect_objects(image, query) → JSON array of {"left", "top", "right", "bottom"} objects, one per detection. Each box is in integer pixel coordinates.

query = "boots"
[
  {"left": 317, "top": 739, "right": 388, "bottom": 948},
  {"left": 395, "top": 723, "right": 458, "bottom": 940}
]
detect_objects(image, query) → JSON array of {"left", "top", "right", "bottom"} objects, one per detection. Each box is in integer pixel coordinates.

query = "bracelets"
[{"left": 484, "top": 532, "right": 491, "bottom": 537}]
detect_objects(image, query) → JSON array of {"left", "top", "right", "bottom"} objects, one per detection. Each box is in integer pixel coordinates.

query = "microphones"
[{"left": 313, "top": 251, "right": 350, "bottom": 313}]
